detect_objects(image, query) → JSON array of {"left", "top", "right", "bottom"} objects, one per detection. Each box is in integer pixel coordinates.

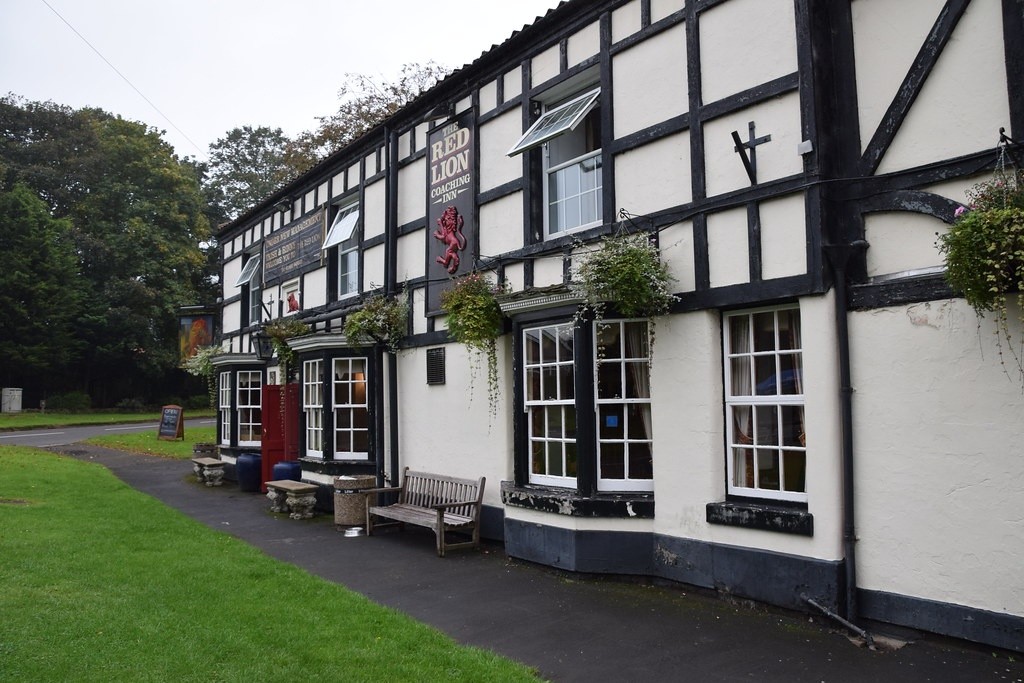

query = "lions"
[
  {"left": 434, "top": 205, "right": 466, "bottom": 275},
  {"left": 286, "top": 294, "right": 300, "bottom": 313}
]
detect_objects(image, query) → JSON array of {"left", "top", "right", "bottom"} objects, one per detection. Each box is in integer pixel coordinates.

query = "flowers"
[
  {"left": 557, "top": 209, "right": 681, "bottom": 394},
  {"left": 179, "top": 342, "right": 233, "bottom": 409},
  {"left": 444, "top": 269, "right": 512, "bottom": 433},
  {"left": 933, "top": 165, "right": 1024, "bottom": 386}
]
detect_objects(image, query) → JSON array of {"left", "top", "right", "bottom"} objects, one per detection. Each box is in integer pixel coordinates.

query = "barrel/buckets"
[
  {"left": 236, "top": 453, "right": 261, "bottom": 492},
  {"left": 273, "top": 461, "right": 302, "bottom": 482}
]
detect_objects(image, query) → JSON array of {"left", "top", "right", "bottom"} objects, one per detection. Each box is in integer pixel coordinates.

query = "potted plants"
[{"left": 342, "top": 272, "right": 409, "bottom": 353}]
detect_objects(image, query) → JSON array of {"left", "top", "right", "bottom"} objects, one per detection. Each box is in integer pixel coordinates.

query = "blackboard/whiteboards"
[{"left": 157, "top": 405, "right": 185, "bottom": 441}]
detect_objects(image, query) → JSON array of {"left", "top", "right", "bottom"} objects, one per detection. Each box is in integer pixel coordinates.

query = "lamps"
[
  {"left": 273, "top": 195, "right": 298, "bottom": 213},
  {"left": 251, "top": 318, "right": 276, "bottom": 360},
  {"left": 423, "top": 102, "right": 456, "bottom": 121}
]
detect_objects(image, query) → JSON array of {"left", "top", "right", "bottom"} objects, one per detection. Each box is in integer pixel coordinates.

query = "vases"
[{"left": 979, "top": 258, "right": 1024, "bottom": 293}]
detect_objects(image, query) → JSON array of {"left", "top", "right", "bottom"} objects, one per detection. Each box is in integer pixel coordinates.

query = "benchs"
[
  {"left": 191, "top": 457, "right": 227, "bottom": 487},
  {"left": 264, "top": 480, "right": 320, "bottom": 520},
  {"left": 358, "top": 466, "right": 486, "bottom": 557}
]
